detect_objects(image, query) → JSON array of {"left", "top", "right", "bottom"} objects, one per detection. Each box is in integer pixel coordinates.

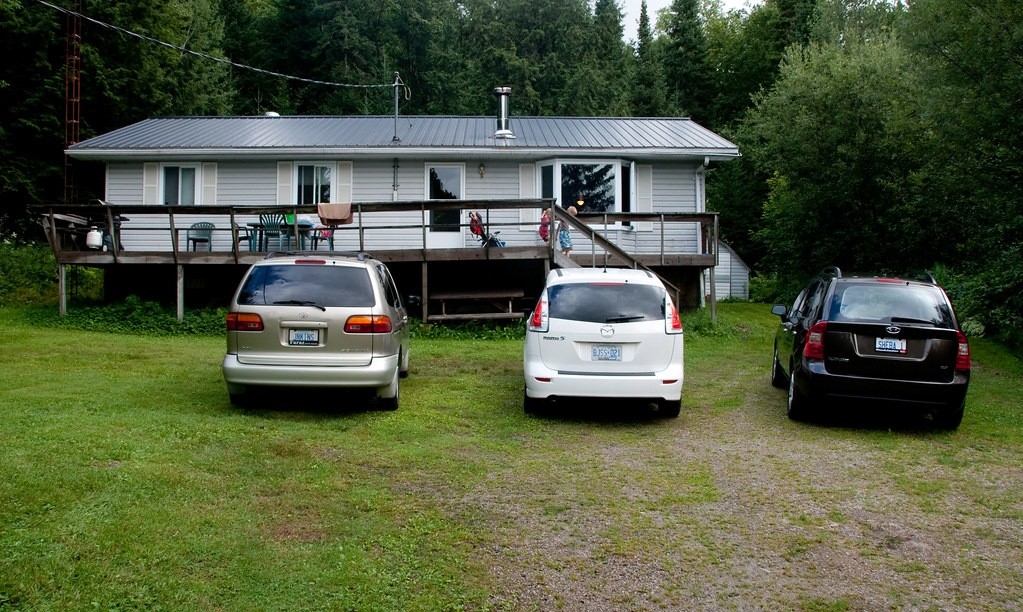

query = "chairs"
[
  {"left": 186, "top": 222, "right": 216, "bottom": 252},
  {"left": 880, "top": 300, "right": 922, "bottom": 324},
  {"left": 233, "top": 209, "right": 335, "bottom": 252}
]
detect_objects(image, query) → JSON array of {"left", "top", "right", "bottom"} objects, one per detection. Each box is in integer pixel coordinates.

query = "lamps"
[
  {"left": 479, "top": 164, "right": 486, "bottom": 177},
  {"left": 576, "top": 196, "right": 585, "bottom": 206}
]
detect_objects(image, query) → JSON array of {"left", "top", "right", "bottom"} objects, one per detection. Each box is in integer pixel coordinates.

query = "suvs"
[
  {"left": 222, "top": 252, "right": 409, "bottom": 411},
  {"left": 770, "top": 266, "right": 970, "bottom": 429}
]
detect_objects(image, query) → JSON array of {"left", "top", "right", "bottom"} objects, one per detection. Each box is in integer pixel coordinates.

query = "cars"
[{"left": 523, "top": 268, "right": 684, "bottom": 418}]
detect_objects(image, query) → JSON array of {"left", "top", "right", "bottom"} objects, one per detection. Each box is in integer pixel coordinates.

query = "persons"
[
  {"left": 555, "top": 206, "right": 577, "bottom": 258},
  {"left": 539, "top": 208, "right": 552, "bottom": 246}
]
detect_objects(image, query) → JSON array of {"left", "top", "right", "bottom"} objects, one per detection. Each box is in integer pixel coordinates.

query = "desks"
[{"left": 247, "top": 223, "right": 314, "bottom": 252}]
